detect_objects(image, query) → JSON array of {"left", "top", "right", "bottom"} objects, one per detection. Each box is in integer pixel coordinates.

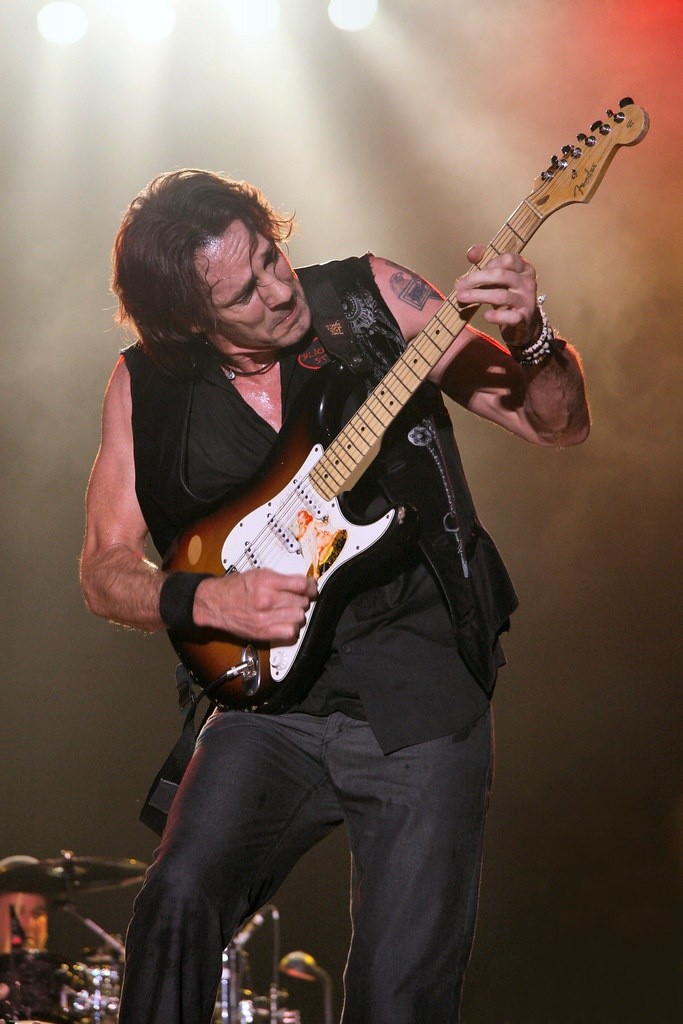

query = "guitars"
[{"left": 162, "top": 96, "right": 650, "bottom": 716}]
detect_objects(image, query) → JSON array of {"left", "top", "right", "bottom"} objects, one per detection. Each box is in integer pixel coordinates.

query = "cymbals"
[{"left": 1, "top": 856, "right": 149, "bottom": 898}]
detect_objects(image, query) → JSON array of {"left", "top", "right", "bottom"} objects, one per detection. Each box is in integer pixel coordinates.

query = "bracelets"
[
  {"left": 160, "top": 573, "right": 213, "bottom": 631},
  {"left": 507, "top": 295, "right": 556, "bottom": 365}
]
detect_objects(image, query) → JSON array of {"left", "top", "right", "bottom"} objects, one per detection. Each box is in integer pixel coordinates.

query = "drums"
[{"left": 0, "top": 940, "right": 301, "bottom": 1024}]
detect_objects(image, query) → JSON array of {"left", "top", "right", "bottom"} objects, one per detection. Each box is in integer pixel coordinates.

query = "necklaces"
[{"left": 218, "top": 354, "right": 278, "bottom": 380}]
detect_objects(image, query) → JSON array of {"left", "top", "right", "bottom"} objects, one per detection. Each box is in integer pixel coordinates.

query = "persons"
[
  {"left": 0, "top": 855, "right": 51, "bottom": 951},
  {"left": 80, "top": 169, "right": 590, "bottom": 1024}
]
